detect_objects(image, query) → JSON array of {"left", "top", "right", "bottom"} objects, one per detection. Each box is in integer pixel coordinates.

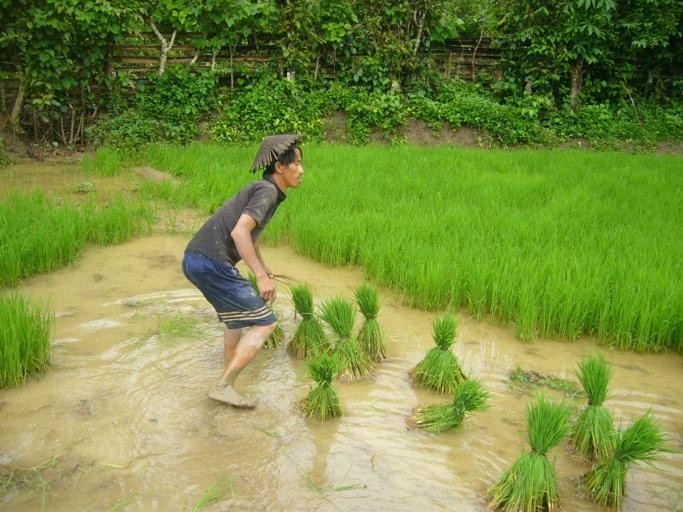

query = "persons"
[{"left": 182, "top": 134, "right": 304, "bottom": 409}]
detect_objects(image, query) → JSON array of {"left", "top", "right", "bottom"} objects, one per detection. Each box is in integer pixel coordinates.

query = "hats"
[{"left": 249, "top": 135, "right": 303, "bottom": 174}]
